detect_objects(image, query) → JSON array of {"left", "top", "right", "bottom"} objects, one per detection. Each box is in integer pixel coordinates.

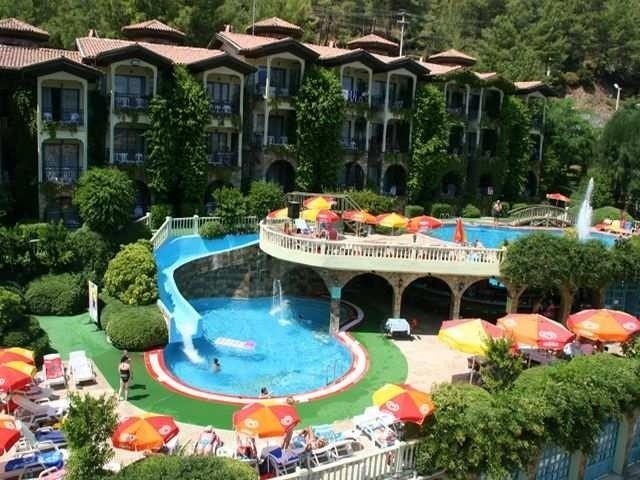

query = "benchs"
[{"left": 0, "top": 452, "right": 61, "bottom": 480}]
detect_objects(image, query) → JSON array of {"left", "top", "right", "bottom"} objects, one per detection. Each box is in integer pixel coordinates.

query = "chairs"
[
  {"left": 294, "top": 218, "right": 314, "bottom": 234},
  {"left": 12, "top": 350, "right": 97, "bottom": 451},
  {"left": 351, "top": 406, "right": 400, "bottom": 449},
  {"left": 261, "top": 425, "right": 359, "bottom": 477},
  {"left": 595, "top": 216, "right": 639, "bottom": 238},
  {"left": 191, "top": 431, "right": 260, "bottom": 469}
]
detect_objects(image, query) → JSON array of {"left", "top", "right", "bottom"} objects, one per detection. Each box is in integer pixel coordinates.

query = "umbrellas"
[
  {"left": 372, "top": 383, "right": 435, "bottom": 442},
  {"left": 546, "top": 193, "right": 570, "bottom": 206},
  {"left": 438, "top": 309, "right": 640, "bottom": 383},
  {"left": 233, "top": 401, "right": 300, "bottom": 472},
  {"left": 111, "top": 413, "right": 179, "bottom": 452},
  {"left": 0, "top": 361, "right": 38, "bottom": 393},
  {"left": 0, "top": 414, "right": 20, "bottom": 456},
  {"left": 0, "top": 347, "right": 35, "bottom": 367},
  {"left": 266, "top": 196, "right": 466, "bottom": 246}
]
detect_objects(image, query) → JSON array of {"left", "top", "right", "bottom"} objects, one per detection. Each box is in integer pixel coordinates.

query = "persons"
[
  {"left": 493, "top": 200, "right": 502, "bottom": 225},
  {"left": 258, "top": 387, "right": 273, "bottom": 398},
  {"left": 211, "top": 358, "right": 222, "bottom": 373},
  {"left": 143, "top": 424, "right": 327, "bottom": 460},
  {"left": 118, "top": 359, "right": 132, "bottom": 401},
  {"left": 461, "top": 240, "right": 487, "bottom": 257},
  {"left": 121, "top": 349, "right": 133, "bottom": 385}
]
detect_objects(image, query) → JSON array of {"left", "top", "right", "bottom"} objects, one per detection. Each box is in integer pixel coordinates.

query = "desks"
[{"left": 386, "top": 317, "right": 411, "bottom": 338}]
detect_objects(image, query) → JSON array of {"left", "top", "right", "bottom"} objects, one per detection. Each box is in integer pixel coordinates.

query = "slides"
[{"left": 153, "top": 232, "right": 260, "bottom": 343}]
